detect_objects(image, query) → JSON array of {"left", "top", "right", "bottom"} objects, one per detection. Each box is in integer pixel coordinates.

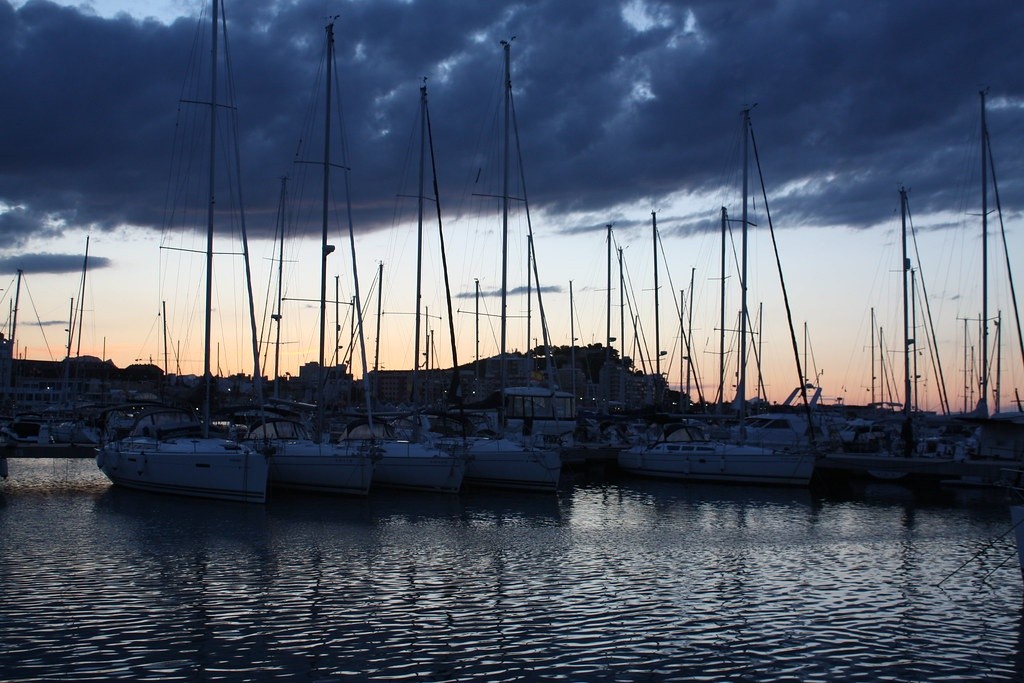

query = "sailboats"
[{"left": 1, "top": 0, "right": 1024, "bottom": 580}]
